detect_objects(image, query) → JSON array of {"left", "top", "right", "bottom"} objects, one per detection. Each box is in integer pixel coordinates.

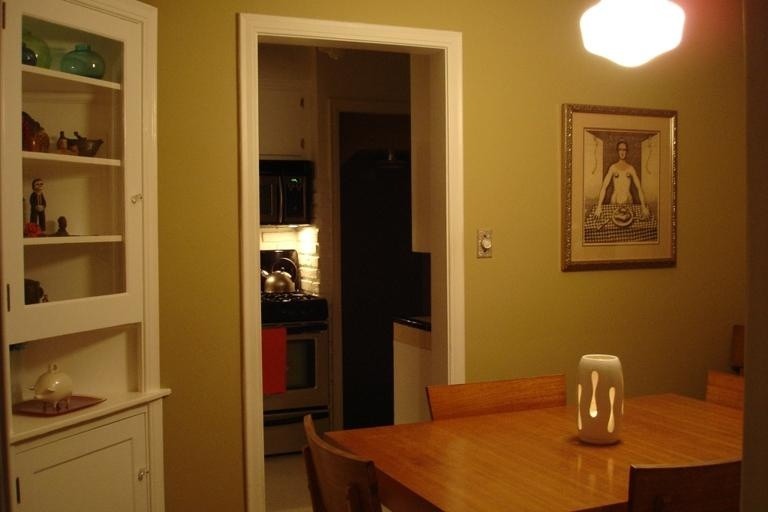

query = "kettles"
[{"left": 260, "top": 258, "right": 300, "bottom": 295}]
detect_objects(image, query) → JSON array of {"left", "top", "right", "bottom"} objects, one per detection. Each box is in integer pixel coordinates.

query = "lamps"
[{"left": 580, "top": 0, "right": 687, "bottom": 70}]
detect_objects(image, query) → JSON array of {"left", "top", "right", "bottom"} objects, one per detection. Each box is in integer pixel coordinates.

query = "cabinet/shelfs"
[{"left": 2, "top": 0, "right": 172, "bottom": 511}]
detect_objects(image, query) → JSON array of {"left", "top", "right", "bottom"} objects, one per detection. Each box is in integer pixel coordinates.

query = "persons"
[
  {"left": 592, "top": 141, "right": 651, "bottom": 224},
  {"left": 29, "top": 178, "right": 46, "bottom": 235}
]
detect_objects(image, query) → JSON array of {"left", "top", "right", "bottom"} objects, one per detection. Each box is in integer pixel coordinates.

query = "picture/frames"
[{"left": 561, "top": 103, "right": 680, "bottom": 272}]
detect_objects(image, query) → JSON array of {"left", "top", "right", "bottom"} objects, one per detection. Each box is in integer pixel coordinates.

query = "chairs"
[
  {"left": 296, "top": 412, "right": 382, "bottom": 509},
  {"left": 704, "top": 368, "right": 744, "bottom": 407},
  {"left": 628, "top": 460, "right": 742, "bottom": 510},
  {"left": 425, "top": 376, "right": 571, "bottom": 418}
]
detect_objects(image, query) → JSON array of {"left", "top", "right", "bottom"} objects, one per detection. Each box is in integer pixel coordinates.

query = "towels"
[{"left": 263, "top": 325, "right": 287, "bottom": 396}]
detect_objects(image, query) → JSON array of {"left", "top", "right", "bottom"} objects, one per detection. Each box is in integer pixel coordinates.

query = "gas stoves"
[{"left": 261, "top": 290, "right": 331, "bottom": 328}]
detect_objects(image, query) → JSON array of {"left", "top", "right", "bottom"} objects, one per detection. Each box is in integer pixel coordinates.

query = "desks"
[{"left": 324, "top": 390, "right": 745, "bottom": 512}]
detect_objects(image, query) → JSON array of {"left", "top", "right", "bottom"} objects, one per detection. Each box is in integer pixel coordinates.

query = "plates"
[{"left": 11, "top": 396, "right": 106, "bottom": 417}]
